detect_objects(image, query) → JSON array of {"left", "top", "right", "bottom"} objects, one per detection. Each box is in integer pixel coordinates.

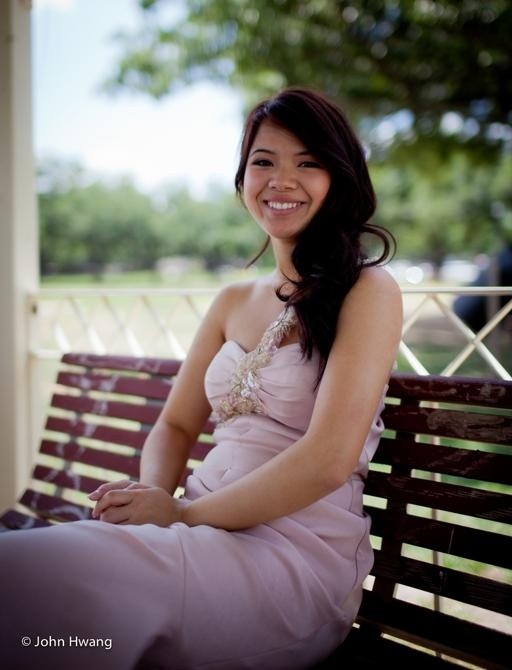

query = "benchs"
[{"left": 0, "top": 350, "right": 512, "bottom": 670}]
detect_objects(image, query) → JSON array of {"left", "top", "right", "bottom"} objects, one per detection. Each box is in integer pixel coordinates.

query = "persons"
[{"left": 0, "top": 87, "right": 404, "bottom": 668}]
